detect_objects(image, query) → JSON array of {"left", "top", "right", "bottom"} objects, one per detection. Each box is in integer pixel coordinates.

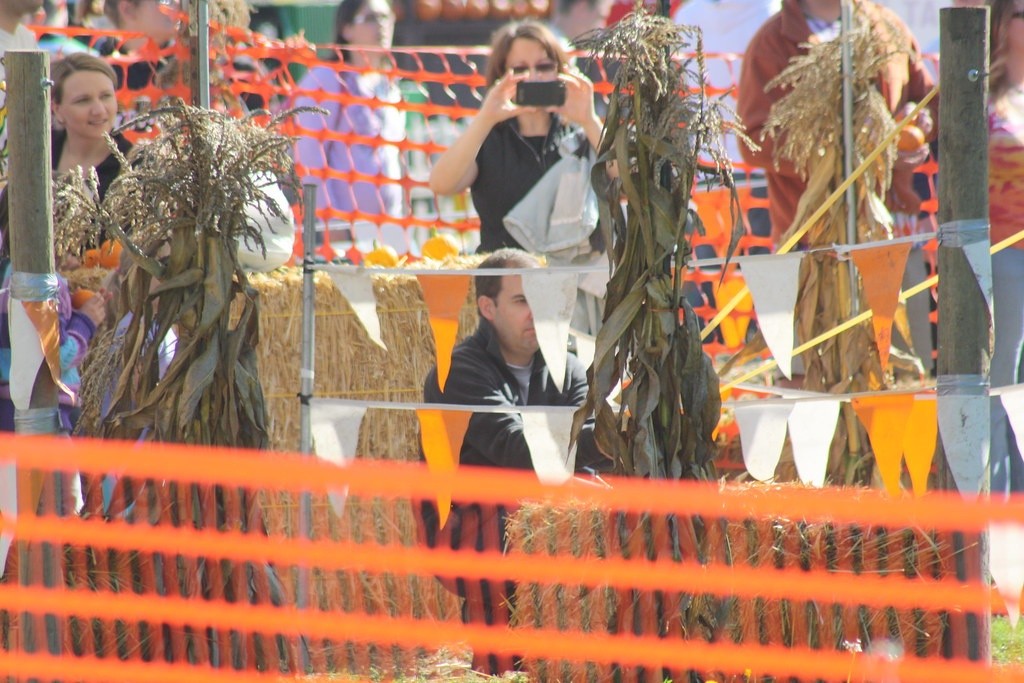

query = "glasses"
[
  {"left": 500, "top": 62, "right": 556, "bottom": 75},
  {"left": 348, "top": 13, "right": 396, "bottom": 24}
]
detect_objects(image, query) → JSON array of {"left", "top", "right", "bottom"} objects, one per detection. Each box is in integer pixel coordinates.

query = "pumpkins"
[
  {"left": 422, "top": 226, "right": 459, "bottom": 261},
  {"left": 71, "top": 239, "right": 121, "bottom": 309},
  {"left": 365, "top": 238, "right": 399, "bottom": 267},
  {"left": 893, "top": 123, "right": 926, "bottom": 153}
]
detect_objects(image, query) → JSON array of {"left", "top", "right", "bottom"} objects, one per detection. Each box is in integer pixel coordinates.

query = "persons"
[
  {"left": 737, "top": 0, "right": 940, "bottom": 392},
  {"left": 987, "top": 1, "right": 1024, "bottom": 388},
  {"left": 429, "top": 23, "right": 618, "bottom": 252},
  {"left": 419, "top": 249, "right": 613, "bottom": 676},
  {"left": 293, "top": 0, "right": 416, "bottom": 252},
  {"left": 0, "top": 1, "right": 293, "bottom": 578}
]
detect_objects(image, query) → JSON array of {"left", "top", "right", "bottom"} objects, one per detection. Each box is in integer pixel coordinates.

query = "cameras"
[{"left": 517, "top": 82, "right": 566, "bottom": 107}]
